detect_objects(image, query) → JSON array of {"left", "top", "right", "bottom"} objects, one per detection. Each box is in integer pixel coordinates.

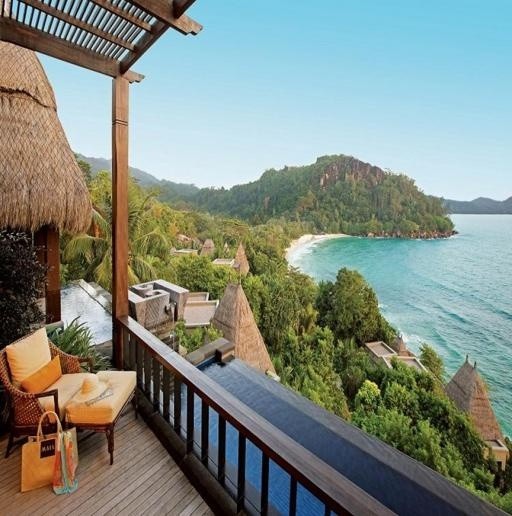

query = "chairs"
[{"left": 0, "top": 325, "right": 94, "bottom": 462}]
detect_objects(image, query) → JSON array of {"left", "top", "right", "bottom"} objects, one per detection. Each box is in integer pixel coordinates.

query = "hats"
[{"left": 73, "top": 374, "right": 108, "bottom": 402}]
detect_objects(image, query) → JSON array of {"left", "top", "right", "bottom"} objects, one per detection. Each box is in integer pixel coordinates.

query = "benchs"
[{"left": 64, "top": 369, "right": 140, "bottom": 467}]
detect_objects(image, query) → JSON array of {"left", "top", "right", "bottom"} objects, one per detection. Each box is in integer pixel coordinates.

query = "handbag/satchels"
[{"left": 21, "top": 411, "right": 79, "bottom": 492}]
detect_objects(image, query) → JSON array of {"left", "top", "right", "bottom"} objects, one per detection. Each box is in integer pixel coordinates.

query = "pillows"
[
  {"left": 4, "top": 325, "right": 53, "bottom": 394},
  {"left": 18, "top": 352, "right": 64, "bottom": 396}
]
